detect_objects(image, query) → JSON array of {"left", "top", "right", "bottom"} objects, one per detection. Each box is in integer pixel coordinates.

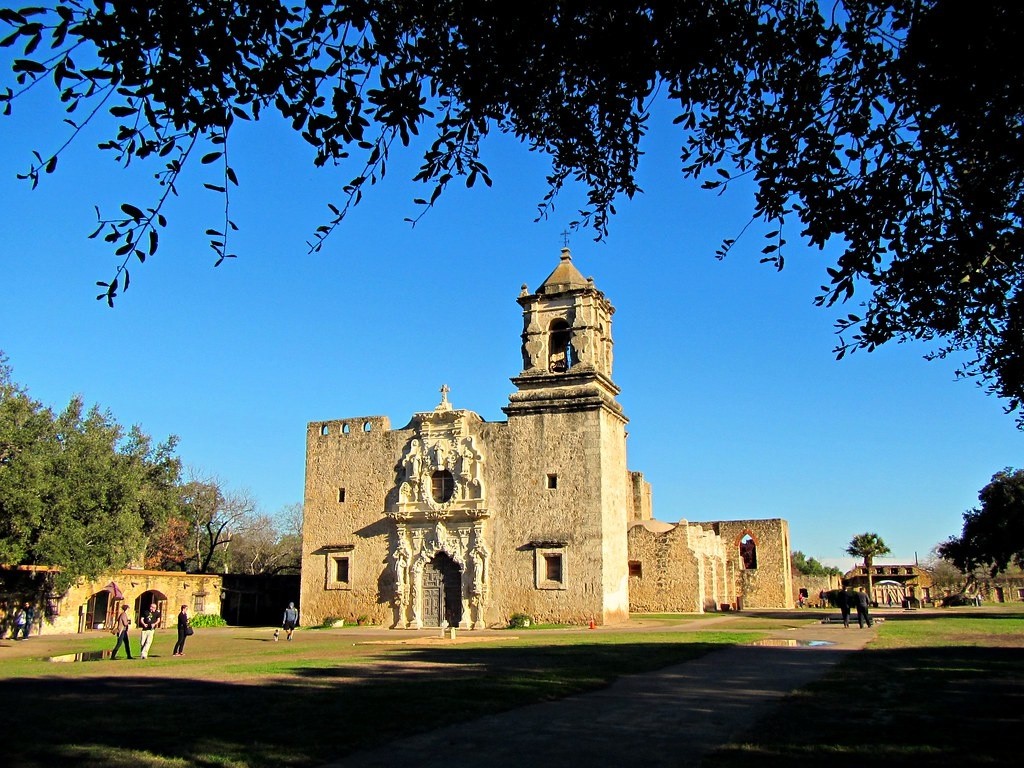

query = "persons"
[
  {"left": 0, "top": 602, "right": 35, "bottom": 641},
  {"left": 140, "top": 603, "right": 161, "bottom": 659},
  {"left": 434, "top": 439, "right": 444, "bottom": 463},
  {"left": 818, "top": 589, "right": 826, "bottom": 609},
  {"left": 887, "top": 591, "right": 894, "bottom": 607},
  {"left": 798, "top": 592, "right": 803, "bottom": 608},
  {"left": 837, "top": 585, "right": 852, "bottom": 628},
  {"left": 435, "top": 521, "right": 446, "bottom": 542},
  {"left": 391, "top": 530, "right": 422, "bottom": 606},
  {"left": 978, "top": 591, "right": 982, "bottom": 606},
  {"left": 110, "top": 604, "right": 137, "bottom": 660},
  {"left": 452, "top": 527, "right": 491, "bottom": 594},
  {"left": 283, "top": 602, "right": 299, "bottom": 641},
  {"left": 173, "top": 605, "right": 190, "bottom": 657},
  {"left": 409, "top": 439, "right": 421, "bottom": 482},
  {"left": 855, "top": 588, "right": 872, "bottom": 629},
  {"left": 459, "top": 447, "right": 473, "bottom": 475}
]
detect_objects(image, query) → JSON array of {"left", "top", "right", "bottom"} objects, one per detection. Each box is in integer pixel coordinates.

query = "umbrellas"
[{"left": 874, "top": 579, "right": 903, "bottom": 592}]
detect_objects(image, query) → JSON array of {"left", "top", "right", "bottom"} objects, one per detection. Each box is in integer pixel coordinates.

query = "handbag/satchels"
[{"left": 187, "top": 627, "right": 194, "bottom": 636}]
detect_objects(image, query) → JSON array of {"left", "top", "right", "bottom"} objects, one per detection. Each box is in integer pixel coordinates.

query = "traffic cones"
[{"left": 588, "top": 614, "right": 596, "bottom": 629}]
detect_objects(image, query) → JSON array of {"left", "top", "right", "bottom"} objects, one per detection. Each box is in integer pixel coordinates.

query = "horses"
[{"left": 819, "top": 590, "right": 871, "bottom": 628}]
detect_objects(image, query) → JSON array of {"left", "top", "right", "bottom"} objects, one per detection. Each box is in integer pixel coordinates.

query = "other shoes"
[
  {"left": 127, "top": 657, "right": 133, "bottom": 659},
  {"left": 173, "top": 652, "right": 181, "bottom": 656},
  {"left": 179, "top": 653, "right": 185, "bottom": 656},
  {"left": 111, "top": 658, "right": 117, "bottom": 660}
]
